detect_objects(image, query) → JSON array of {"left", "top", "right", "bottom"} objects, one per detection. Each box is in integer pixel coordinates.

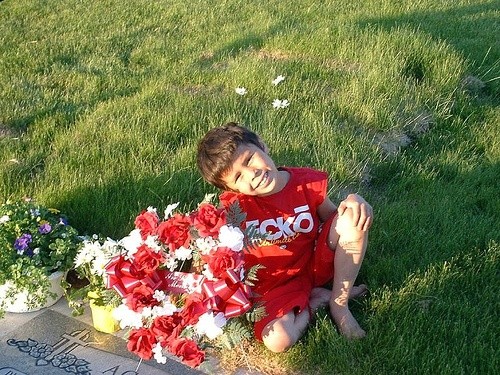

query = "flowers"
[
  {"left": 0, "top": 192, "right": 76, "bottom": 311},
  {"left": 75, "top": 237, "right": 122, "bottom": 306},
  {"left": 103, "top": 194, "right": 264, "bottom": 370}
]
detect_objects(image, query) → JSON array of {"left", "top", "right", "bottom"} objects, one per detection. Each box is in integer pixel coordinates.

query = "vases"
[
  {"left": 0, "top": 268, "right": 70, "bottom": 314},
  {"left": 87, "top": 289, "right": 122, "bottom": 334}
]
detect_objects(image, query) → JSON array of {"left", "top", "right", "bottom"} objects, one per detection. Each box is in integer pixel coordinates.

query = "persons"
[{"left": 194, "top": 121, "right": 372, "bottom": 354}]
